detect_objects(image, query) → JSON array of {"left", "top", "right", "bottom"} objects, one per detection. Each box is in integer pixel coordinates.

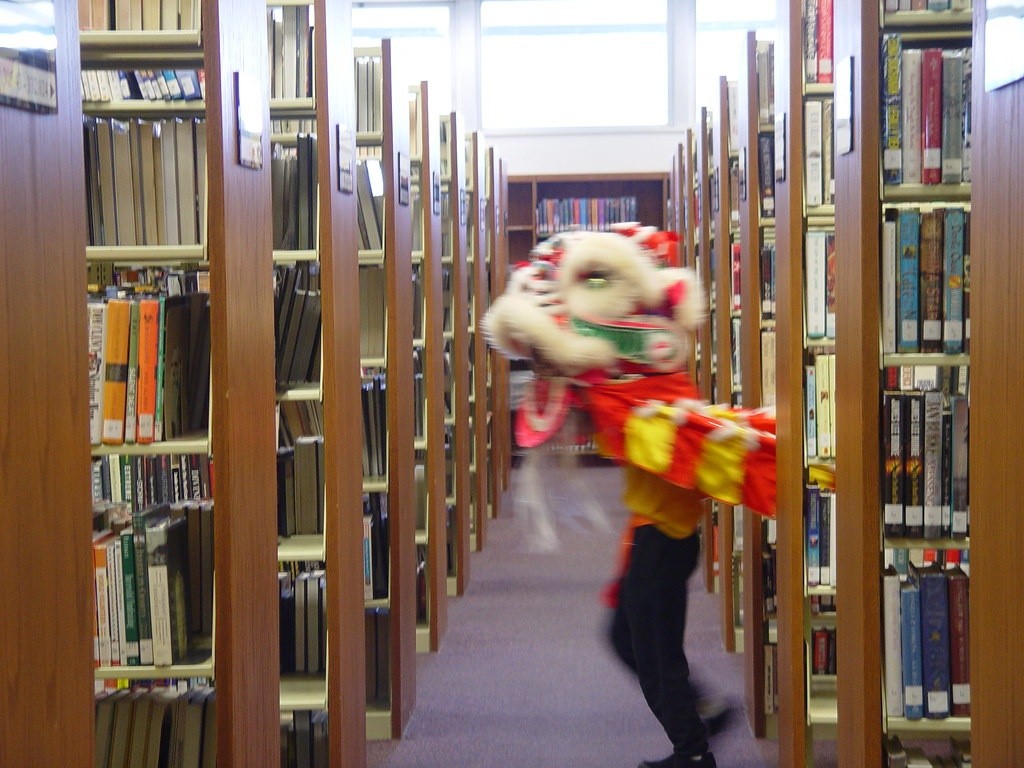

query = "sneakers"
[{"left": 638, "top": 751, "right": 717, "bottom": 768}]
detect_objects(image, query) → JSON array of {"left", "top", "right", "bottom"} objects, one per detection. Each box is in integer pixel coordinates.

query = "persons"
[{"left": 524, "top": 350, "right": 717, "bottom": 768}]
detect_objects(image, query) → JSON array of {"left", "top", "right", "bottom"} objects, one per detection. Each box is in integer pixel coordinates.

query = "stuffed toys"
[{"left": 479, "top": 220, "right": 779, "bottom": 521}]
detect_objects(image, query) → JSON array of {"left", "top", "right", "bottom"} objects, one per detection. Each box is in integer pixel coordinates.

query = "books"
[
  {"left": 667, "top": 0, "right": 836, "bottom": 717},
  {"left": 539, "top": 198, "right": 639, "bottom": 452},
  {"left": 879, "top": 1, "right": 974, "bottom": 768},
  {"left": 268, "top": 3, "right": 502, "bottom": 768},
  {"left": 76, "top": 0, "right": 214, "bottom": 768}
]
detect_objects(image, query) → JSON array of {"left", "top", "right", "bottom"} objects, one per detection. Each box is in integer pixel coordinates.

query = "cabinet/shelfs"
[
  {"left": 507, "top": 172, "right": 672, "bottom": 266},
  {"left": 0, "top": 0, "right": 512, "bottom": 768},
  {"left": 672, "top": 1, "right": 1024, "bottom": 768}
]
